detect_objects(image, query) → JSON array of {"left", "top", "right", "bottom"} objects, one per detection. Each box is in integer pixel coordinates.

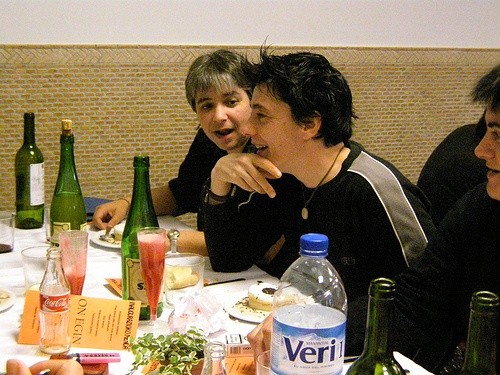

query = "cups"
[
  {"left": 164, "top": 252, "right": 205, "bottom": 307},
  {"left": 114, "top": 224, "right": 125, "bottom": 244},
  {"left": 57, "top": 230, "right": 88, "bottom": 295},
  {"left": 256, "top": 349, "right": 270, "bottom": 375},
  {"left": 43, "top": 203, "right": 52, "bottom": 242},
  {"left": 21, "top": 247, "right": 50, "bottom": 289},
  {"left": 0, "top": 212, "right": 16, "bottom": 253}
]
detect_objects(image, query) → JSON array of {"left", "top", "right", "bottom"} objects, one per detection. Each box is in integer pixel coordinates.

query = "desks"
[{"left": 0, "top": 206, "right": 434, "bottom": 375}]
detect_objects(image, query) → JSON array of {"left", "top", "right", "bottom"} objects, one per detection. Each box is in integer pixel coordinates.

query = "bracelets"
[
  {"left": 119, "top": 197, "right": 130, "bottom": 206},
  {"left": 202, "top": 176, "right": 231, "bottom": 200}
]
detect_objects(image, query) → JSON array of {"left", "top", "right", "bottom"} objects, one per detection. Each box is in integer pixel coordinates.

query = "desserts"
[
  {"left": 113, "top": 223, "right": 126, "bottom": 241},
  {"left": 248, "top": 281, "right": 293, "bottom": 312}
]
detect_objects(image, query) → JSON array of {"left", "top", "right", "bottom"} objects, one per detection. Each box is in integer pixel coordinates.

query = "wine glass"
[{"left": 134, "top": 227, "right": 167, "bottom": 330}]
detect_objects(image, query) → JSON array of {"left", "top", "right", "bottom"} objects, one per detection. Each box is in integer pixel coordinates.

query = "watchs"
[{"left": 168, "top": 228, "right": 180, "bottom": 253}]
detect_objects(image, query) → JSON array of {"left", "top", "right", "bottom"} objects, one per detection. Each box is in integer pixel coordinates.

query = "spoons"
[
  {"left": 100, "top": 224, "right": 113, "bottom": 243},
  {"left": 262, "top": 287, "right": 278, "bottom": 295}
]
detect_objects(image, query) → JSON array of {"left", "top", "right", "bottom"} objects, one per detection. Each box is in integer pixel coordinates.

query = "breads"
[{"left": 171, "top": 267, "right": 197, "bottom": 288}]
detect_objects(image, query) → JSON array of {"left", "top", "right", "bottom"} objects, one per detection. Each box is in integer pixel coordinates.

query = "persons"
[
  {"left": 202, "top": 34, "right": 436, "bottom": 302},
  {"left": 248, "top": 62, "right": 500, "bottom": 375},
  {"left": 6, "top": 358, "right": 84, "bottom": 375},
  {"left": 93, "top": 49, "right": 285, "bottom": 263}
]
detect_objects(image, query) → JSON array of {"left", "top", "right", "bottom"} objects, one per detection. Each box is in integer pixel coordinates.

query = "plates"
[
  {"left": 90, "top": 231, "right": 126, "bottom": 248},
  {"left": 0, "top": 287, "right": 16, "bottom": 313},
  {"left": 222, "top": 288, "right": 274, "bottom": 325}
]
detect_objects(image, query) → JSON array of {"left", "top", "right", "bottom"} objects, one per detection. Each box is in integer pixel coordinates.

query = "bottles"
[
  {"left": 39, "top": 248, "right": 71, "bottom": 355},
  {"left": 50, "top": 120, "right": 86, "bottom": 247},
  {"left": 456, "top": 290, "right": 500, "bottom": 375},
  {"left": 15, "top": 112, "right": 45, "bottom": 228},
  {"left": 268, "top": 234, "right": 347, "bottom": 375},
  {"left": 121, "top": 156, "right": 164, "bottom": 321},
  {"left": 200, "top": 341, "right": 228, "bottom": 375},
  {"left": 345, "top": 278, "right": 407, "bottom": 375}
]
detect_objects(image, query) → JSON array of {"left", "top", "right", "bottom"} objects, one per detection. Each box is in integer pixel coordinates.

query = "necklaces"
[{"left": 300, "top": 146, "right": 346, "bottom": 219}]
